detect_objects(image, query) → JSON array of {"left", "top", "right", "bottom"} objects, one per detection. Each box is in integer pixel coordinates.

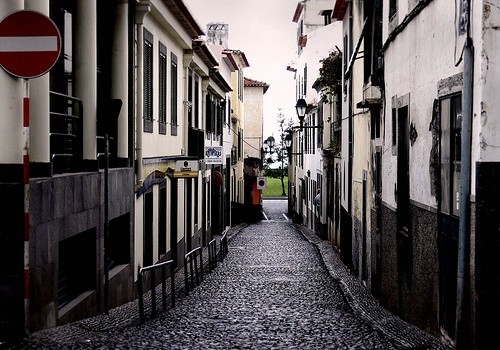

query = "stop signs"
[{"left": 0, "top": 10, "right": 63, "bottom": 79}]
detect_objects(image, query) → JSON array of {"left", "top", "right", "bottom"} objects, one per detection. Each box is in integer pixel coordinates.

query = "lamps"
[{"left": 295, "top": 95, "right": 323, "bottom": 130}]
